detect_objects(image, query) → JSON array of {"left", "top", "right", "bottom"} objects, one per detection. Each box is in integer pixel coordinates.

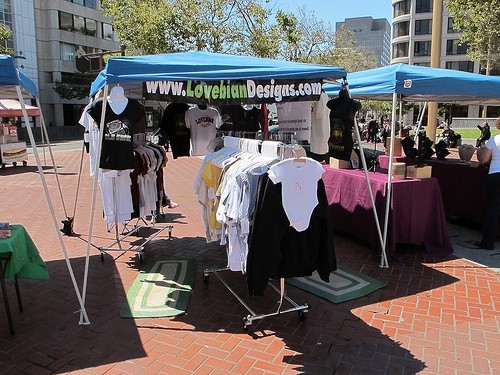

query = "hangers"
[
  {"left": 208, "top": 135, "right": 310, "bottom": 166},
  {"left": 100, "top": 131, "right": 151, "bottom": 147}
]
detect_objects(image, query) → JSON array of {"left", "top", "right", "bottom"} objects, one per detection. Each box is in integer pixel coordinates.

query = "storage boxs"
[
  {"left": 386, "top": 137, "right": 403, "bottom": 156},
  {"left": 328, "top": 156, "right": 352, "bottom": 168},
  {"left": 392, "top": 163, "right": 433, "bottom": 179}
]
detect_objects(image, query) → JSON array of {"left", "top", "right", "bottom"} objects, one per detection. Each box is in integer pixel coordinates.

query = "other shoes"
[
  {"left": 369, "top": 142, "right": 373, "bottom": 145},
  {"left": 475, "top": 242, "right": 493, "bottom": 250},
  {"left": 364, "top": 140, "right": 368, "bottom": 143}
]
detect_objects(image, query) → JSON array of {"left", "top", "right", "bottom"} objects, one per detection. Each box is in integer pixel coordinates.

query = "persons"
[
  {"left": 354, "top": 115, "right": 456, "bottom": 148},
  {"left": 326, "top": 89, "right": 362, "bottom": 161},
  {"left": 474, "top": 117, "right": 500, "bottom": 250}
]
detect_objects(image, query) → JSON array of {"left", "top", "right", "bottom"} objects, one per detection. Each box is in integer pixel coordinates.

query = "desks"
[
  {"left": 0, "top": 224, "right": 49, "bottom": 336},
  {"left": 378, "top": 155, "right": 500, "bottom": 240},
  {"left": 322, "top": 162, "right": 455, "bottom": 263}
]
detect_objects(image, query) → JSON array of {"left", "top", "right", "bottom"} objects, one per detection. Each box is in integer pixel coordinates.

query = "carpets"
[
  {"left": 284, "top": 263, "right": 388, "bottom": 305},
  {"left": 121, "top": 257, "right": 197, "bottom": 318}
]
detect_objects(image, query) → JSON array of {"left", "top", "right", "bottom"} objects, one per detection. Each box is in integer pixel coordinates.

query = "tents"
[
  {"left": 0, "top": 54, "right": 90, "bottom": 325},
  {"left": 321, "top": 62, "right": 500, "bottom": 268},
  {"left": 78, "top": 50, "right": 389, "bottom": 325}
]
